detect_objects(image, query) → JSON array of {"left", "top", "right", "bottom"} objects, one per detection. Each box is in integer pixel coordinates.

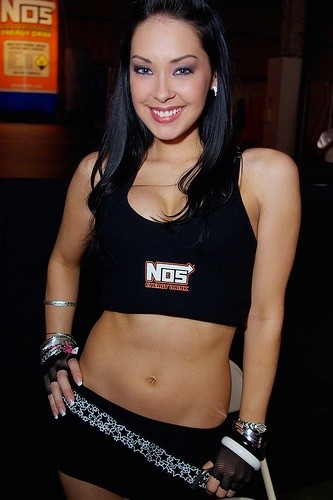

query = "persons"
[{"left": 36, "top": 1, "right": 303, "bottom": 500}]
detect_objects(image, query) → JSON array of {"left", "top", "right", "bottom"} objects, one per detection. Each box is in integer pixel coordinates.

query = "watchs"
[{"left": 236, "top": 419, "right": 268, "bottom": 434}]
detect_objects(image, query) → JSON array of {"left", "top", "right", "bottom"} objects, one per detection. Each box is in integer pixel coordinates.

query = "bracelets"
[
  {"left": 233, "top": 423, "right": 265, "bottom": 447},
  {"left": 39, "top": 332, "right": 77, "bottom": 357},
  {"left": 42, "top": 301, "right": 77, "bottom": 307}
]
